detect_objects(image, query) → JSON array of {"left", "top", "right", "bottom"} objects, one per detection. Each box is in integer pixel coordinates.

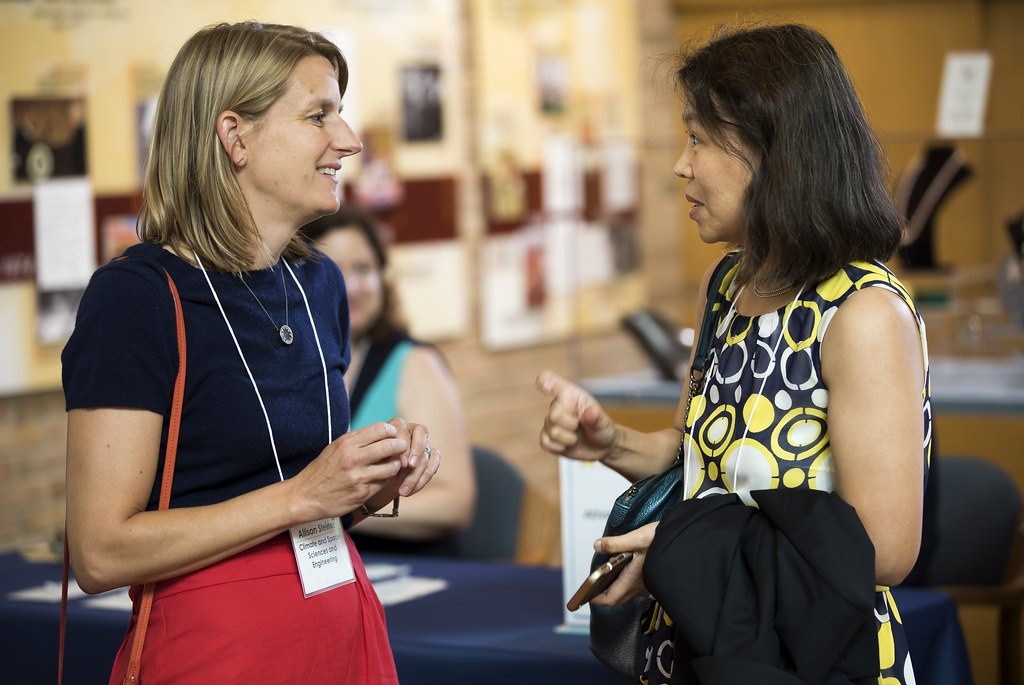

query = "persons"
[
  {"left": 535, "top": 23, "right": 933, "bottom": 685},
  {"left": 886, "top": 141, "right": 973, "bottom": 278},
  {"left": 62, "top": 20, "right": 439, "bottom": 685},
  {"left": 294, "top": 206, "right": 474, "bottom": 554}
]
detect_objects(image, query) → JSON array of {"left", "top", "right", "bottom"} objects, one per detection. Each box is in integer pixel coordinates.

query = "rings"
[{"left": 423, "top": 447, "right": 431, "bottom": 457}]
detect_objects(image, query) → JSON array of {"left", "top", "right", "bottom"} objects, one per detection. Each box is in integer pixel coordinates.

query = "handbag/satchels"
[{"left": 589, "top": 248, "right": 745, "bottom": 678}]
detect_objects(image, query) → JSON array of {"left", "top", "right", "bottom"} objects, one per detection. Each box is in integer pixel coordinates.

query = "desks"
[{"left": 0, "top": 541, "right": 975, "bottom": 685}]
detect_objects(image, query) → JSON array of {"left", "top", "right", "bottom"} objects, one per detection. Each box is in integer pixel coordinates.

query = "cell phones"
[{"left": 567, "top": 552, "right": 633, "bottom": 612}]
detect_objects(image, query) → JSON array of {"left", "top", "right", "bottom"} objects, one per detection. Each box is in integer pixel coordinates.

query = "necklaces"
[
  {"left": 751, "top": 273, "right": 796, "bottom": 298},
  {"left": 235, "top": 267, "right": 293, "bottom": 345},
  {"left": 898, "top": 148, "right": 966, "bottom": 248}
]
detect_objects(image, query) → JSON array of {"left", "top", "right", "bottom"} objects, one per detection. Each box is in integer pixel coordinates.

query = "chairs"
[
  {"left": 437, "top": 444, "right": 532, "bottom": 567},
  {"left": 915, "top": 456, "right": 1022, "bottom": 585}
]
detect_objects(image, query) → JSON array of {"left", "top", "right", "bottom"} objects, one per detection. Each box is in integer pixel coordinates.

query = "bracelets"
[{"left": 359, "top": 496, "right": 399, "bottom": 518}]
